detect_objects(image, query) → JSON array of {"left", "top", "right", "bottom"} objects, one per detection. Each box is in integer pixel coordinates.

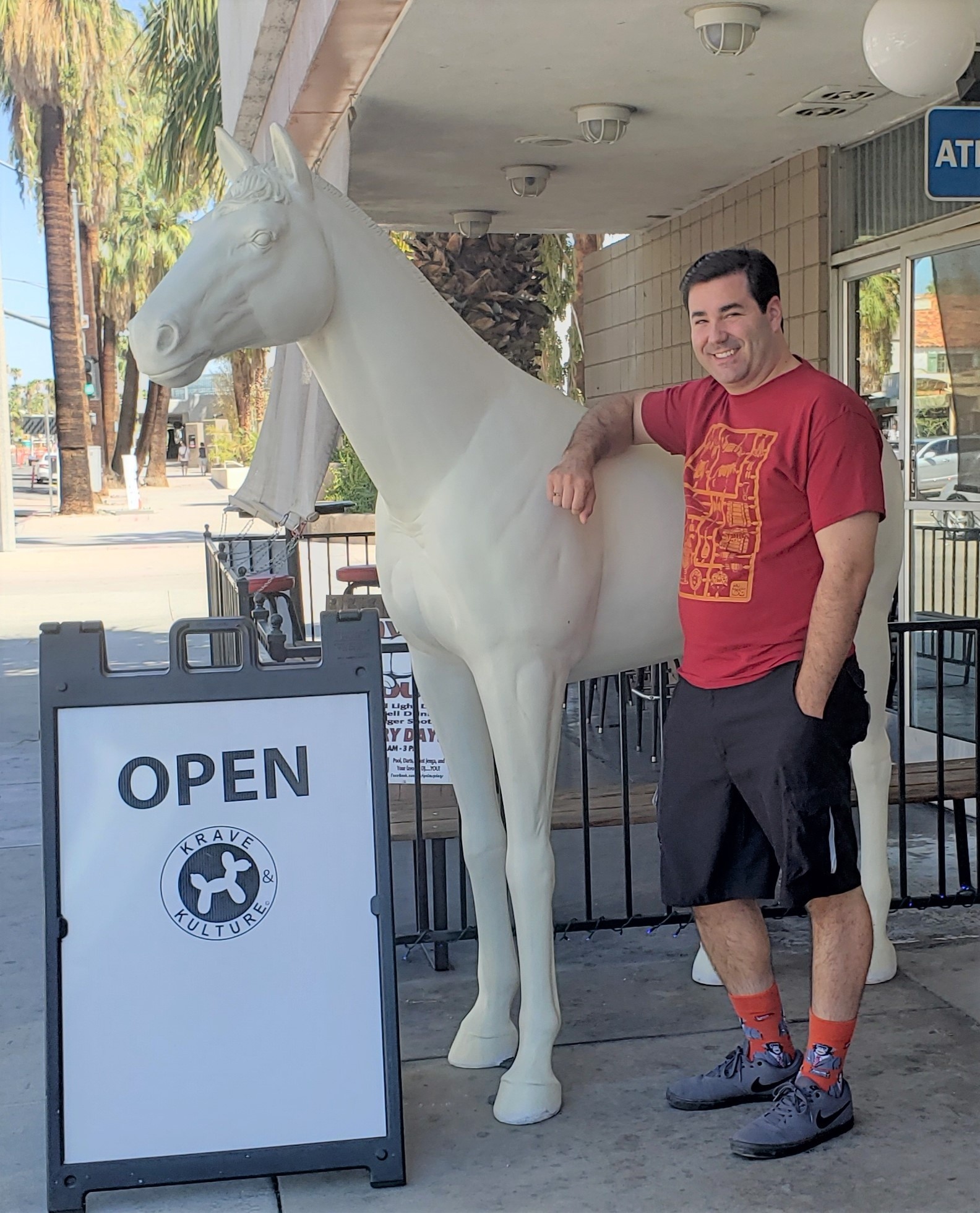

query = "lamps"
[
  {"left": 569, "top": 103, "right": 637, "bottom": 145},
  {"left": 684, "top": 3, "right": 772, "bottom": 56},
  {"left": 501, "top": 163, "right": 556, "bottom": 198},
  {"left": 449, "top": 209, "right": 499, "bottom": 239},
  {"left": 861, "top": 0, "right": 980, "bottom": 102}
]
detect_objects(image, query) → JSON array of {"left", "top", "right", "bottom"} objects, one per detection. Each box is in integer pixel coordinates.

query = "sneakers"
[
  {"left": 730, "top": 1071, "right": 854, "bottom": 1159},
  {"left": 665, "top": 1038, "right": 803, "bottom": 1110}
]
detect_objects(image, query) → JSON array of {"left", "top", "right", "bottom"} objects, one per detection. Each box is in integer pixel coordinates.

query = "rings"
[{"left": 554, "top": 494, "right": 563, "bottom": 496}]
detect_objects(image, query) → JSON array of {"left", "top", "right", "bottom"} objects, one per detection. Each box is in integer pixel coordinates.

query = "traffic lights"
[{"left": 82, "top": 353, "right": 103, "bottom": 403}]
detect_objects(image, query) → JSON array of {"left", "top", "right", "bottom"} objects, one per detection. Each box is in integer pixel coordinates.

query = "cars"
[
  {"left": 36, "top": 452, "right": 60, "bottom": 485},
  {"left": 934, "top": 453, "right": 980, "bottom": 541},
  {"left": 886, "top": 435, "right": 980, "bottom": 499}
]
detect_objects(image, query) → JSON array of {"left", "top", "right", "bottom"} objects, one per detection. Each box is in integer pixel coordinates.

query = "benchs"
[{"left": 389, "top": 757, "right": 980, "bottom": 972}]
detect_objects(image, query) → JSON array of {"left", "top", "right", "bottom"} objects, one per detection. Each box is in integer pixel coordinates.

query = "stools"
[
  {"left": 247, "top": 574, "right": 306, "bottom": 641},
  {"left": 337, "top": 565, "right": 380, "bottom": 595}
]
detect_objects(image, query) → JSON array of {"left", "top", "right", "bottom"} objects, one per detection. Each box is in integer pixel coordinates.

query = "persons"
[
  {"left": 178, "top": 441, "right": 190, "bottom": 476},
  {"left": 197, "top": 443, "right": 209, "bottom": 477},
  {"left": 545, "top": 247, "right": 885, "bottom": 1158}
]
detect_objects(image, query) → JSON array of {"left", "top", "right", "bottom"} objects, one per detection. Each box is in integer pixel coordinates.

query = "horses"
[{"left": 127, "top": 120, "right": 905, "bottom": 1113}]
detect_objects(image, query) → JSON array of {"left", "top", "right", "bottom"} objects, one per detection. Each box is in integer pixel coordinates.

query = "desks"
[{"left": 223, "top": 500, "right": 357, "bottom": 641}]
